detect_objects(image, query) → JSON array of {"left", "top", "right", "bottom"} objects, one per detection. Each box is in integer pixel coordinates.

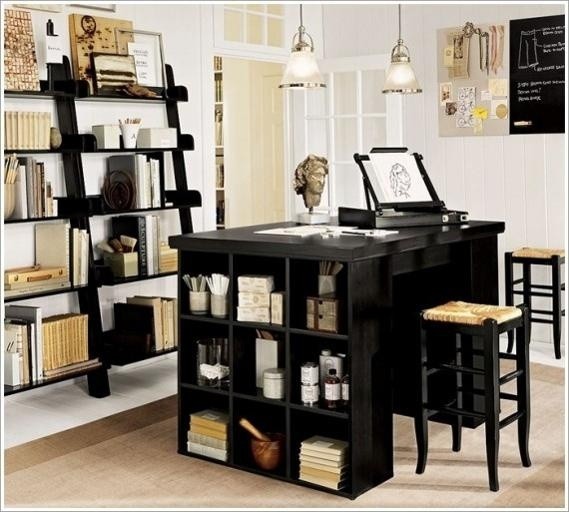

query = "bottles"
[
  {"left": 262, "top": 368, "right": 285, "bottom": 400},
  {"left": 323, "top": 368, "right": 339, "bottom": 411},
  {"left": 340, "top": 373, "right": 351, "bottom": 407}
]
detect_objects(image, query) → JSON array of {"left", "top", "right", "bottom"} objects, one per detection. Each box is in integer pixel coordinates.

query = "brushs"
[{"left": 97, "top": 235, "right": 137, "bottom": 252}]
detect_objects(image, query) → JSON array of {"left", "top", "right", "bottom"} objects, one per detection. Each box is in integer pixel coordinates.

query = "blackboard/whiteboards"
[{"left": 436, "top": 14, "right": 563, "bottom": 137}]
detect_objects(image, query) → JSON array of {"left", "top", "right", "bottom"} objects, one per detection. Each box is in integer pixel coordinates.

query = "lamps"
[{"left": 278, "top": 4, "right": 423, "bottom": 95}]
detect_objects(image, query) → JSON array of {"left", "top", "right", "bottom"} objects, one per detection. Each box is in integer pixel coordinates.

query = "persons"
[{"left": 292, "top": 152, "right": 329, "bottom": 208}]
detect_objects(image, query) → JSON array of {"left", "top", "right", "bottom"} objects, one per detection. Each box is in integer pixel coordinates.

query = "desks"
[{"left": 169, "top": 212, "right": 504, "bottom": 501}]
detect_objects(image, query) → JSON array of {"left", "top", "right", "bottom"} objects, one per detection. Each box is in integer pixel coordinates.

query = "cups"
[
  {"left": 210, "top": 294, "right": 227, "bottom": 318},
  {"left": 318, "top": 273, "right": 337, "bottom": 296},
  {"left": 188, "top": 290, "right": 210, "bottom": 316},
  {"left": 119, "top": 124, "right": 141, "bottom": 149}
]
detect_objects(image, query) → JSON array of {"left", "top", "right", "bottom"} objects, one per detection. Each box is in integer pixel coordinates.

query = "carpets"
[{"left": 5, "top": 360, "right": 565, "bottom": 508}]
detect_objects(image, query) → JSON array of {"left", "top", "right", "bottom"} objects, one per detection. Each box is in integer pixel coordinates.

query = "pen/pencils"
[
  {"left": 5, "top": 155, "right": 19, "bottom": 182},
  {"left": 342, "top": 231, "right": 365, "bottom": 235},
  {"left": 118, "top": 118, "right": 140, "bottom": 123}
]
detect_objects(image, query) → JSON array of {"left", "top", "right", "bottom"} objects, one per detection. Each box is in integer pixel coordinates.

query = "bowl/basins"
[{"left": 251, "top": 433, "right": 283, "bottom": 470}]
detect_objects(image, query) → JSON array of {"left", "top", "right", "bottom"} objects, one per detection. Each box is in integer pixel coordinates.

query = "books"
[
  {"left": 3, "top": 305, "right": 101, "bottom": 385},
  {"left": 298, "top": 433, "right": 351, "bottom": 491},
  {"left": 35, "top": 223, "right": 89, "bottom": 287},
  {"left": 269, "top": 290, "right": 287, "bottom": 327},
  {"left": 183, "top": 406, "right": 231, "bottom": 466},
  {"left": 237, "top": 291, "right": 271, "bottom": 309},
  {"left": 114, "top": 294, "right": 180, "bottom": 352},
  {"left": 4, "top": 111, "right": 52, "bottom": 150},
  {"left": 236, "top": 306, "right": 271, "bottom": 323},
  {"left": 10, "top": 156, "right": 54, "bottom": 220},
  {"left": 236, "top": 274, "right": 279, "bottom": 293},
  {"left": 111, "top": 214, "right": 180, "bottom": 275},
  {"left": 109, "top": 153, "right": 165, "bottom": 208}
]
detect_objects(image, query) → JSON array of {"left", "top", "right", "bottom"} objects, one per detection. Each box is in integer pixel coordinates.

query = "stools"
[
  {"left": 504, "top": 247, "right": 565, "bottom": 359},
  {"left": 414, "top": 301, "right": 531, "bottom": 492}
]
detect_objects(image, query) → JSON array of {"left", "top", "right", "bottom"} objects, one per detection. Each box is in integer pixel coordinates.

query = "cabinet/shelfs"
[
  {"left": 3, "top": 60, "right": 108, "bottom": 396},
  {"left": 58, "top": 56, "right": 203, "bottom": 396},
  {"left": 214, "top": 57, "right": 225, "bottom": 229}
]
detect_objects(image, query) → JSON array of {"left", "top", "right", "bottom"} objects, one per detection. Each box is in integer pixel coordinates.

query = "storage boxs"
[
  {"left": 102, "top": 249, "right": 141, "bottom": 279},
  {"left": 135, "top": 124, "right": 180, "bottom": 148},
  {"left": 91, "top": 124, "right": 121, "bottom": 149}
]
